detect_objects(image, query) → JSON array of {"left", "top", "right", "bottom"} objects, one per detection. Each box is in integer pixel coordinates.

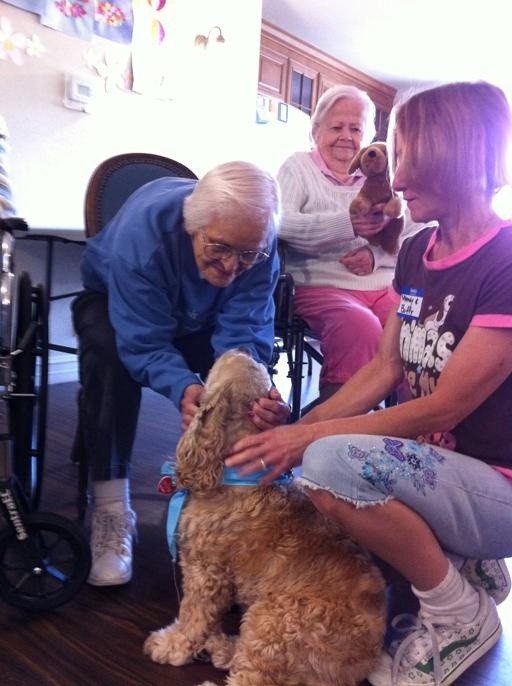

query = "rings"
[{"left": 259, "top": 458, "right": 266, "bottom": 469}]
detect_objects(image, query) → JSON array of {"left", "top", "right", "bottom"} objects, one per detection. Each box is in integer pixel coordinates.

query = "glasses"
[{"left": 199, "top": 228, "right": 270, "bottom": 265}]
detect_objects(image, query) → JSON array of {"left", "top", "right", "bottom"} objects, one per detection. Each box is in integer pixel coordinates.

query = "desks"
[{"left": 2, "top": 228, "right": 86, "bottom": 508}]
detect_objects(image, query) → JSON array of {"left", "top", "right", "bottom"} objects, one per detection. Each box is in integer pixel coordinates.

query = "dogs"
[{"left": 143, "top": 345, "right": 390, "bottom": 686}]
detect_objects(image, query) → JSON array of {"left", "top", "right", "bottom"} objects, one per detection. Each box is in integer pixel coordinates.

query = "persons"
[
  {"left": 275, "top": 85, "right": 415, "bottom": 405},
  {"left": 223, "top": 79, "right": 512, "bottom": 686},
  {"left": 70, "top": 160, "right": 281, "bottom": 585}
]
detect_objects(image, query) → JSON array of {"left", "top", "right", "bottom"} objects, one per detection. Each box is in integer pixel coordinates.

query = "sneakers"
[
  {"left": 87, "top": 506, "right": 137, "bottom": 588},
  {"left": 366, "top": 558, "right": 511, "bottom": 686}
]
